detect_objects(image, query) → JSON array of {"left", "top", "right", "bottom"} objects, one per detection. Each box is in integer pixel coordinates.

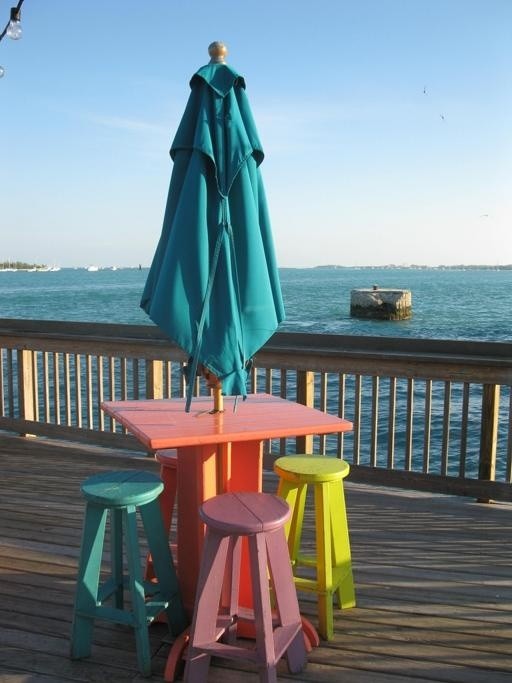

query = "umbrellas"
[{"left": 136, "top": 40, "right": 285, "bottom": 411}]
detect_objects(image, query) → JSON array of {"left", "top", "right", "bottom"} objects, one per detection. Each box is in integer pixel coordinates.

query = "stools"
[
  {"left": 267, "top": 452, "right": 355, "bottom": 639},
  {"left": 143, "top": 450, "right": 185, "bottom": 593},
  {"left": 78, "top": 469, "right": 190, "bottom": 681},
  {"left": 185, "top": 491, "right": 310, "bottom": 683}
]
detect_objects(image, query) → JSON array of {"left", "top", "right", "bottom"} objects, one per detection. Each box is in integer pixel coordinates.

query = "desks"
[{"left": 98, "top": 392, "right": 354, "bottom": 637}]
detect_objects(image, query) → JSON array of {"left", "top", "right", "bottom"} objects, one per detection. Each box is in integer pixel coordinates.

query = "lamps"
[{"left": 5, "top": 8, "right": 25, "bottom": 41}]
